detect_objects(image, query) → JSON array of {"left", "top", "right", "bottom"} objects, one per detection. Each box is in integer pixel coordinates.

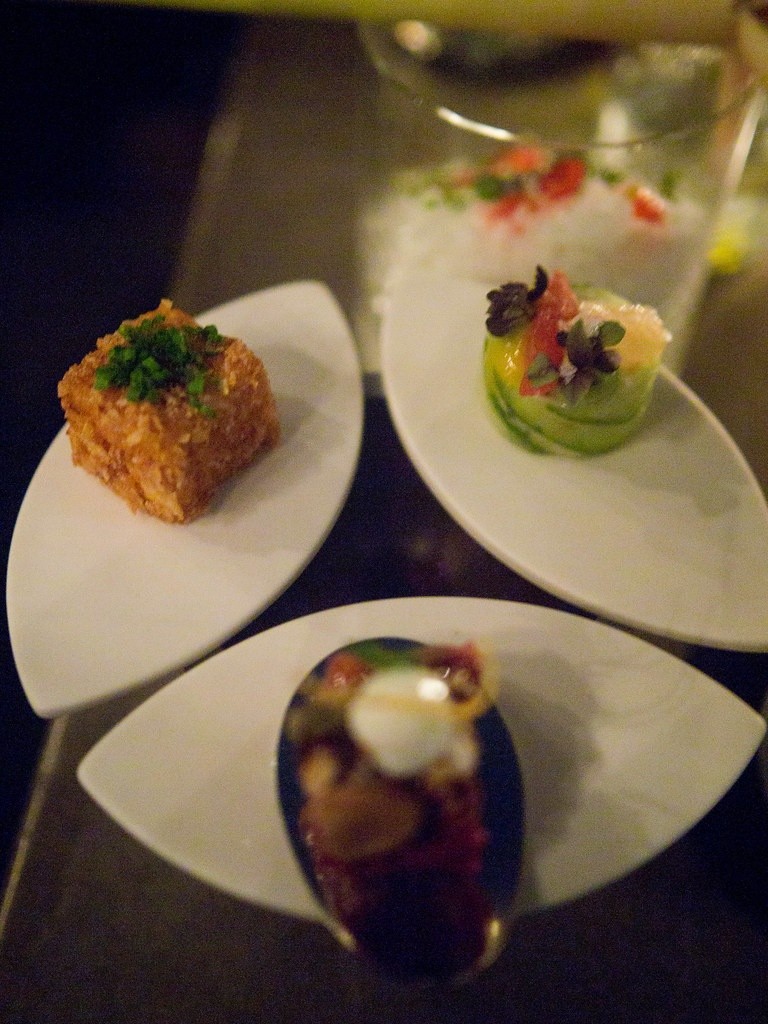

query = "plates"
[
  {"left": 6, "top": 281, "right": 364, "bottom": 719},
  {"left": 379, "top": 267, "right": 768, "bottom": 655},
  {"left": 75, "top": 597, "right": 765, "bottom": 922}
]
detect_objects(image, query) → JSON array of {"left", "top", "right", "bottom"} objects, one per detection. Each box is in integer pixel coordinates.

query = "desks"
[{"left": 0, "top": 14, "right": 768, "bottom": 1024}]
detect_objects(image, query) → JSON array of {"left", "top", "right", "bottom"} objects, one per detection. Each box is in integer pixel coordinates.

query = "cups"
[{"left": 348, "top": 2, "right": 768, "bottom": 383}]
